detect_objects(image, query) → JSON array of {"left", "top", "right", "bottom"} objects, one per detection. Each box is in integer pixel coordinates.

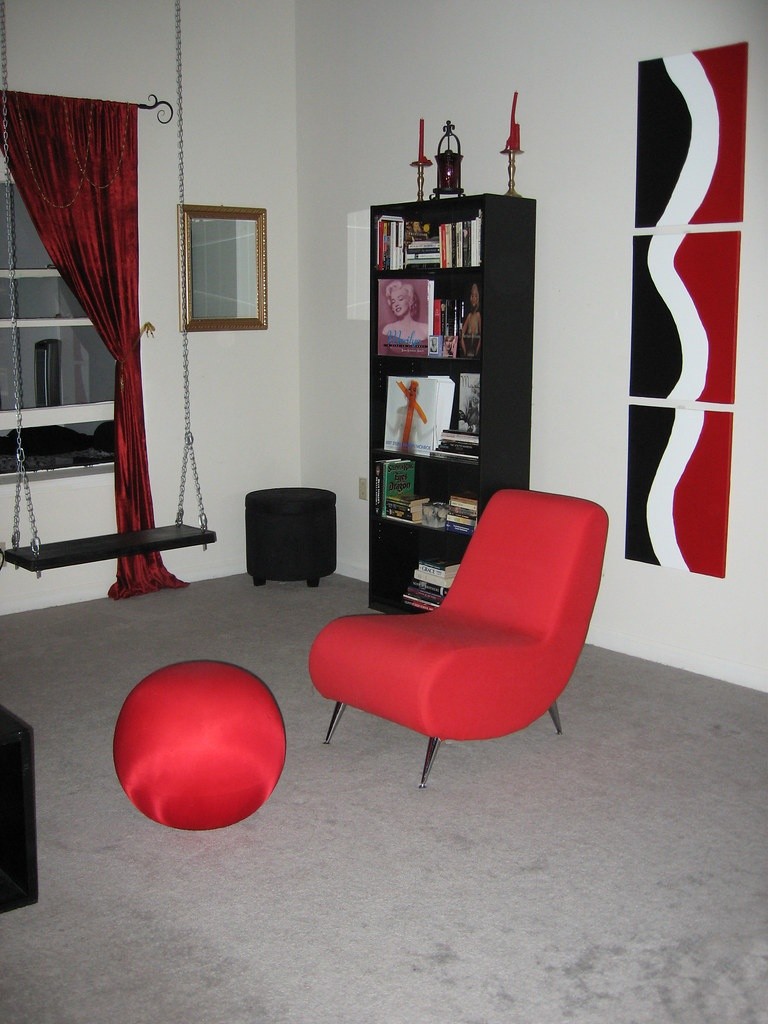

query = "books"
[
  {"left": 378, "top": 279, "right": 434, "bottom": 357},
  {"left": 402, "top": 556, "right": 461, "bottom": 612},
  {"left": 375, "top": 373, "right": 478, "bottom": 536},
  {"left": 376, "top": 215, "right": 482, "bottom": 270},
  {"left": 432, "top": 299, "right": 459, "bottom": 336}
]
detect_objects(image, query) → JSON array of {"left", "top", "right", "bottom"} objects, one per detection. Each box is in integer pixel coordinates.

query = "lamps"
[{"left": 429, "top": 120, "right": 466, "bottom": 200}]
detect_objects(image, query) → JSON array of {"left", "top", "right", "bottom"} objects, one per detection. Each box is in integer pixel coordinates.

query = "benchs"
[{"left": 0, "top": 0, "right": 219, "bottom": 573}]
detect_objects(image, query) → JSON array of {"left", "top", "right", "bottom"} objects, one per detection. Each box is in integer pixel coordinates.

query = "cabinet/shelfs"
[{"left": 368, "top": 193, "right": 537, "bottom": 617}]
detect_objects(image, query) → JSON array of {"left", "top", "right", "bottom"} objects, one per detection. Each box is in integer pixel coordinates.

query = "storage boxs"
[{"left": 1, "top": 704, "right": 38, "bottom": 914}]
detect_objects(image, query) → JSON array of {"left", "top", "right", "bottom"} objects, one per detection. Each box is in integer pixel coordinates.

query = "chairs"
[{"left": 308, "top": 489, "right": 610, "bottom": 789}]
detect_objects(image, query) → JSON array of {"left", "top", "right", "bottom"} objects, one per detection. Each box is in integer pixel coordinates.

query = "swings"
[{"left": 0, "top": 0, "right": 217, "bottom": 570}]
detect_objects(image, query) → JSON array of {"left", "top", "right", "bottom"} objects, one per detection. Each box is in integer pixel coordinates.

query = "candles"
[
  {"left": 418, "top": 116, "right": 431, "bottom": 164},
  {"left": 505, "top": 90, "right": 521, "bottom": 151}
]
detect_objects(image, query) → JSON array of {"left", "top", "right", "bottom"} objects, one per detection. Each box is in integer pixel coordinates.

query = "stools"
[{"left": 244, "top": 487, "right": 337, "bottom": 587}]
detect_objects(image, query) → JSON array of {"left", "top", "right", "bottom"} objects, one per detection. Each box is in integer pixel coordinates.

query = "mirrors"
[{"left": 177, "top": 204, "right": 268, "bottom": 331}]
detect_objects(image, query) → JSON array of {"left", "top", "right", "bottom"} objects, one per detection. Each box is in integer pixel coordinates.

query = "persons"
[
  {"left": 460, "top": 284, "right": 482, "bottom": 358},
  {"left": 444, "top": 336, "right": 456, "bottom": 358},
  {"left": 429, "top": 339, "right": 437, "bottom": 352}
]
